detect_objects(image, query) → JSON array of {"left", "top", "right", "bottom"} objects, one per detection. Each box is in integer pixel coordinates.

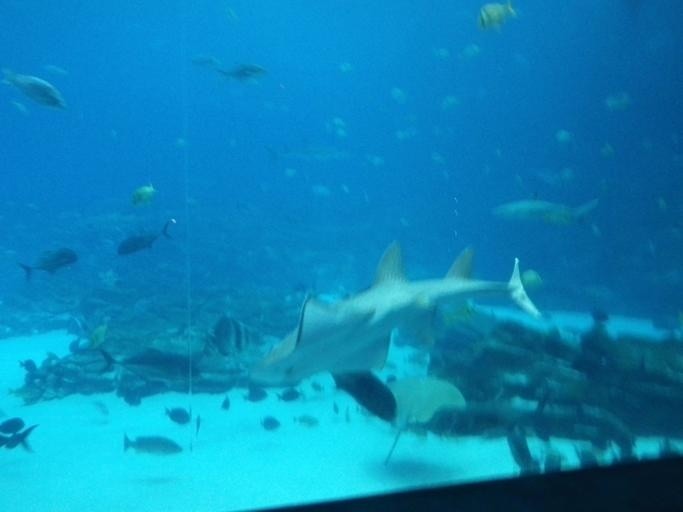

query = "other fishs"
[
  {"left": 190, "top": 41, "right": 481, "bottom": 202},
  {"left": 0, "top": 310, "right": 466, "bottom": 473}
]
